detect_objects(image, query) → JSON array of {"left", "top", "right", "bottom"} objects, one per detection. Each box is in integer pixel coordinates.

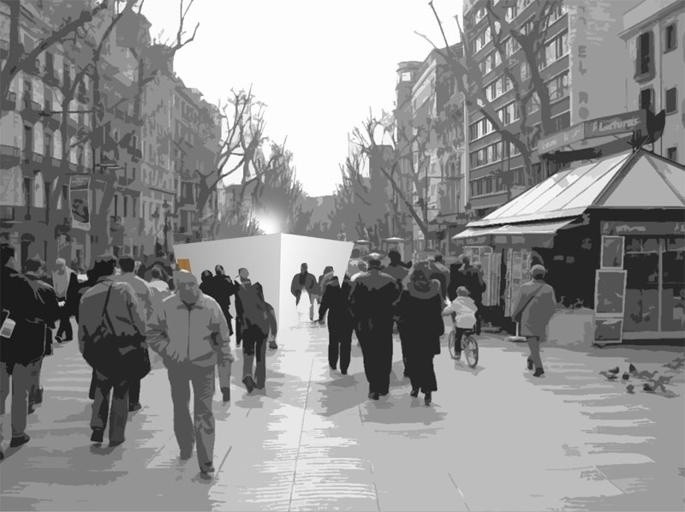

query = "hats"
[{"left": 530, "top": 263, "right": 547, "bottom": 278}]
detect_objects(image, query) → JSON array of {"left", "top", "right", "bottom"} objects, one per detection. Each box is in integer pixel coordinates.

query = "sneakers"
[
  {"left": 129, "top": 402, "right": 142, "bottom": 411},
  {"left": 424, "top": 393, "right": 433, "bottom": 405},
  {"left": 181, "top": 441, "right": 195, "bottom": 461},
  {"left": 329, "top": 362, "right": 350, "bottom": 376},
  {"left": 90, "top": 431, "right": 104, "bottom": 442},
  {"left": 410, "top": 389, "right": 421, "bottom": 397},
  {"left": 220, "top": 374, "right": 267, "bottom": 401},
  {"left": 533, "top": 367, "right": 544, "bottom": 376},
  {"left": 28, "top": 391, "right": 44, "bottom": 414},
  {"left": 526, "top": 359, "right": 534, "bottom": 371},
  {"left": 55, "top": 334, "right": 73, "bottom": 343},
  {"left": 11, "top": 433, "right": 30, "bottom": 447},
  {"left": 110, "top": 440, "right": 125, "bottom": 446},
  {"left": 199, "top": 462, "right": 215, "bottom": 473},
  {"left": 367, "top": 388, "right": 389, "bottom": 400}
]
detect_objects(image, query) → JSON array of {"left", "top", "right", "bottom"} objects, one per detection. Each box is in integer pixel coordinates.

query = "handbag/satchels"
[
  {"left": 0, "top": 312, "right": 47, "bottom": 365},
  {"left": 103, "top": 337, "right": 152, "bottom": 381}
]
